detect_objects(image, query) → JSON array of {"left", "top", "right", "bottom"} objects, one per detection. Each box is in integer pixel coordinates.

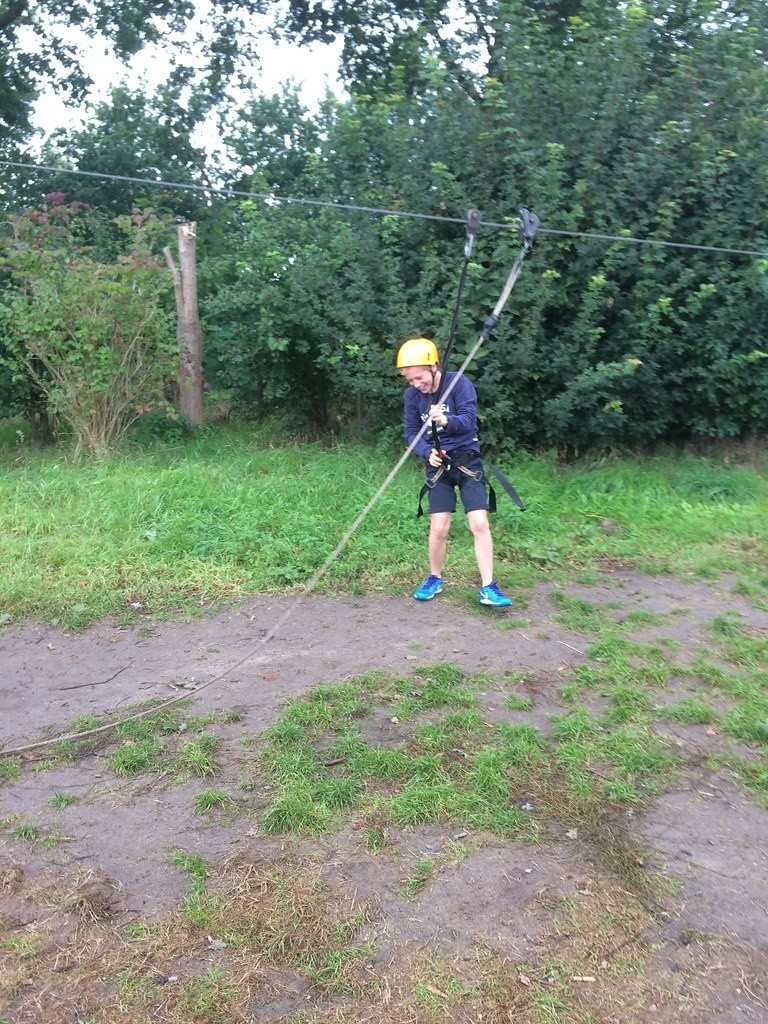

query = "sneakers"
[
  {"left": 479, "top": 581, "right": 513, "bottom": 607},
  {"left": 414, "top": 574, "right": 443, "bottom": 601}
]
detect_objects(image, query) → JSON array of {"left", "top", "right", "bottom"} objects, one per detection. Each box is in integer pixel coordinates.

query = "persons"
[{"left": 396, "top": 339, "right": 512, "bottom": 608}]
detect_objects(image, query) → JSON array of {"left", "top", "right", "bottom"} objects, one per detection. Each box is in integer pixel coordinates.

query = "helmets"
[{"left": 397, "top": 338, "right": 439, "bottom": 368}]
